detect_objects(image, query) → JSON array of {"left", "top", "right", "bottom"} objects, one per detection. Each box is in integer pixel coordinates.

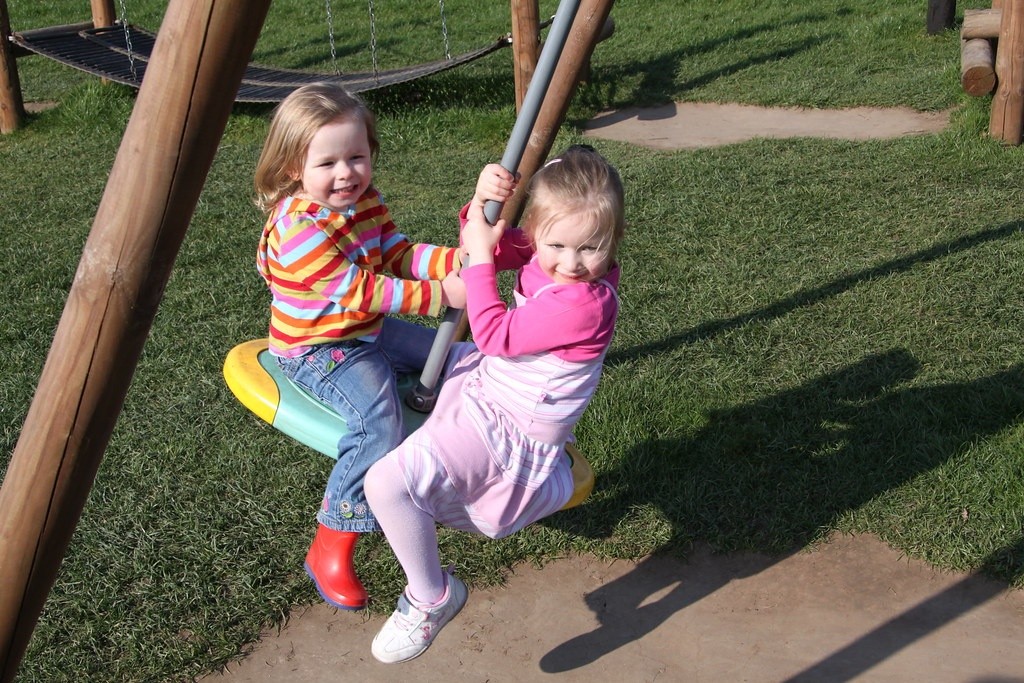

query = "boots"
[{"left": 303, "top": 521, "right": 368, "bottom": 612}]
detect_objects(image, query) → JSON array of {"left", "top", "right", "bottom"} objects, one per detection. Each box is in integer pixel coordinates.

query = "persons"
[
  {"left": 250, "top": 80, "right": 469, "bottom": 613},
  {"left": 351, "top": 143, "right": 625, "bottom": 666}
]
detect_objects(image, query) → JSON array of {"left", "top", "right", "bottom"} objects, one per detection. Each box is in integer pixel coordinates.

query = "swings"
[{"left": 221, "top": 0, "right": 618, "bottom": 511}]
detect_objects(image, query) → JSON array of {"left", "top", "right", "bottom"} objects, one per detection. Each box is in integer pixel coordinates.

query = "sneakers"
[{"left": 371, "top": 563, "right": 469, "bottom": 666}]
